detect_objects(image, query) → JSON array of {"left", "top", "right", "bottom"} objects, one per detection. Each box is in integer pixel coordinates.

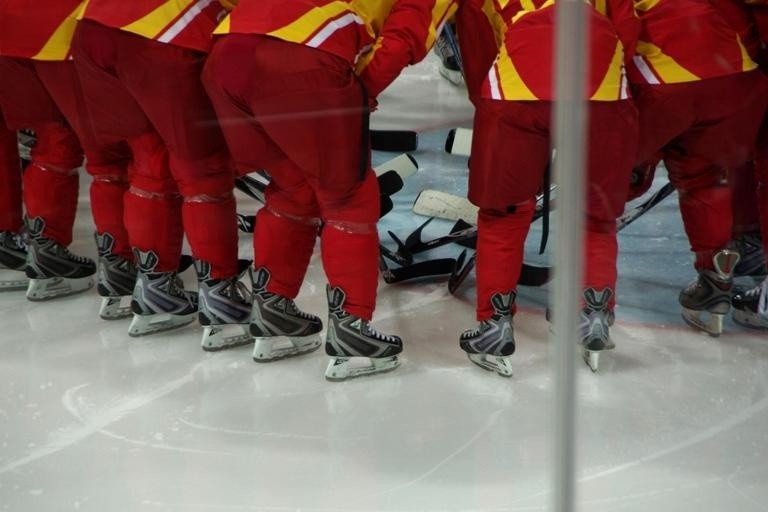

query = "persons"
[
  {"left": 621, "top": 1, "right": 766, "bottom": 314},
  {"left": 199, "top": 1, "right": 463, "bottom": 359},
  {"left": 724, "top": 0, "right": 768, "bottom": 317},
  {"left": 1, "top": 0, "right": 30, "bottom": 273},
  {"left": 0, "top": 0, "right": 135, "bottom": 299},
  {"left": 451, "top": 2, "right": 642, "bottom": 356},
  {"left": 74, "top": 1, "right": 257, "bottom": 328}
]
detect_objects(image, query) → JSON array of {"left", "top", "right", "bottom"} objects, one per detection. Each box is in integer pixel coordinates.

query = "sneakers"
[
  {"left": 325, "top": 283, "right": 402, "bottom": 358},
  {"left": 95, "top": 232, "right": 137, "bottom": 295},
  {"left": 679, "top": 229, "right": 768, "bottom": 321},
  {"left": 132, "top": 246, "right": 198, "bottom": 315},
  {"left": 247, "top": 264, "right": 322, "bottom": 336},
  {"left": 0, "top": 230, "right": 28, "bottom": 271},
  {"left": 546, "top": 286, "right": 615, "bottom": 350},
  {"left": 24, "top": 214, "right": 96, "bottom": 279},
  {"left": 191, "top": 259, "right": 252, "bottom": 325},
  {"left": 460, "top": 290, "right": 517, "bottom": 355}
]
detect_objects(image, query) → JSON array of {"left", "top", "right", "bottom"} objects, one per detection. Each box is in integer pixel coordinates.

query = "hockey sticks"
[
  {"left": 245, "top": 153, "right": 419, "bottom": 182},
  {"left": 516, "top": 181, "right": 677, "bottom": 287},
  {"left": 367, "top": 129, "right": 419, "bottom": 152},
  {"left": 444, "top": 129, "right": 556, "bottom": 163},
  {"left": 231, "top": 170, "right": 479, "bottom": 293},
  {"left": 231, "top": 128, "right": 677, "bottom": 294},
  {"left": 414, "top": 186, "right": 562, "bottom": 227}
]
